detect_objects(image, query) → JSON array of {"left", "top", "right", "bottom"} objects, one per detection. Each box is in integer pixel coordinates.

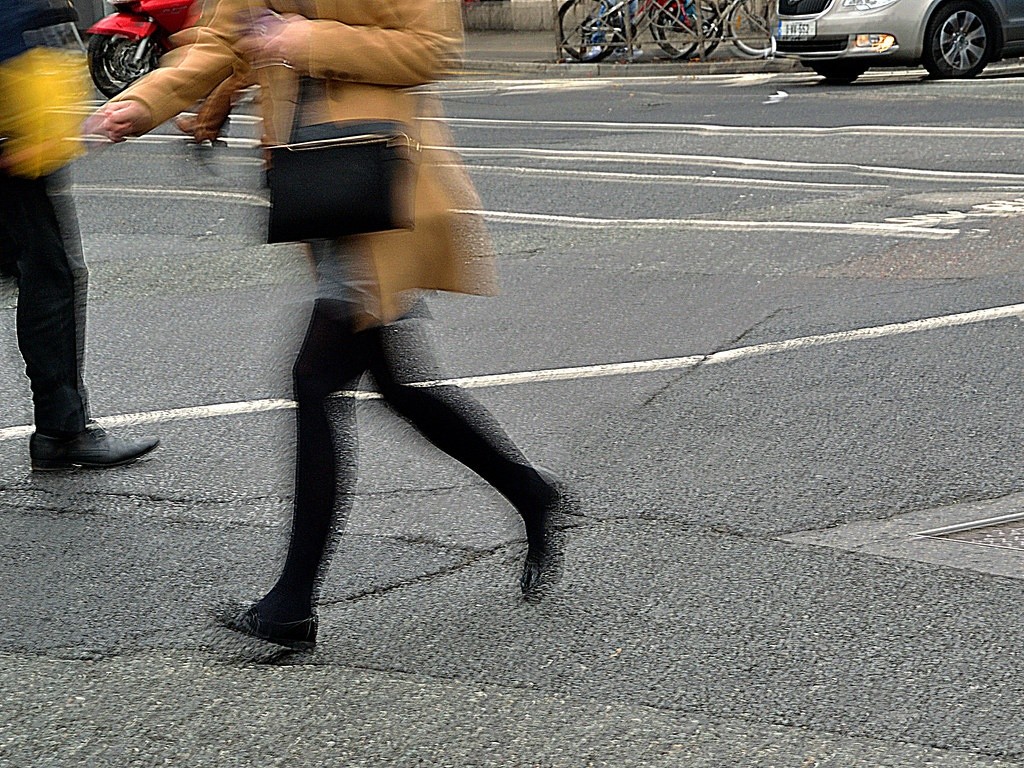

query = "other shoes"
[
  {"left": 518, "top": 488, "right": 573, "bottom": 603},
  {"left": 216, "top": 600, "right": 319, "bottom": 650}
]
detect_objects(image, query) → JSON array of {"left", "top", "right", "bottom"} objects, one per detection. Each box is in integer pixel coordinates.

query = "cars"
[{"left": 765, "top": 0, "right": 1024, "bottom": 80}]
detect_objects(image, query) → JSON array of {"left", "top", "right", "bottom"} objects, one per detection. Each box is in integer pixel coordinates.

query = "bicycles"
[{"left": 558, "top": 0, "right": 779, "bottom": 63}]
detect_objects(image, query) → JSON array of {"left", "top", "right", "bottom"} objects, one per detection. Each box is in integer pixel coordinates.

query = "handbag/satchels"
[
  {"left": 1, "top": 43, "right": 94, "bottom": 179},
  {"left": 261, "top": 118, "right": 422, "bottom": 244}
]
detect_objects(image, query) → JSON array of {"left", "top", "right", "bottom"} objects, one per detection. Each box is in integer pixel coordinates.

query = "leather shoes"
[{"left": 30, "top": 420, "right": 160, "bottom": 472}]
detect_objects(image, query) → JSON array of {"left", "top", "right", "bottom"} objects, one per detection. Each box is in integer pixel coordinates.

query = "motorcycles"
[{"left": 85, "top": 0, "right": 199, "bottom": 100}]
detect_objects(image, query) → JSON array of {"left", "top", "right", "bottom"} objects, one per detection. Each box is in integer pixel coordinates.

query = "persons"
[
  {"left": 0, "top": 0, "right": 160, "bottom": 472},
  {"left": 81, "top": 0, "right": 567, "bottom": 653}
]
